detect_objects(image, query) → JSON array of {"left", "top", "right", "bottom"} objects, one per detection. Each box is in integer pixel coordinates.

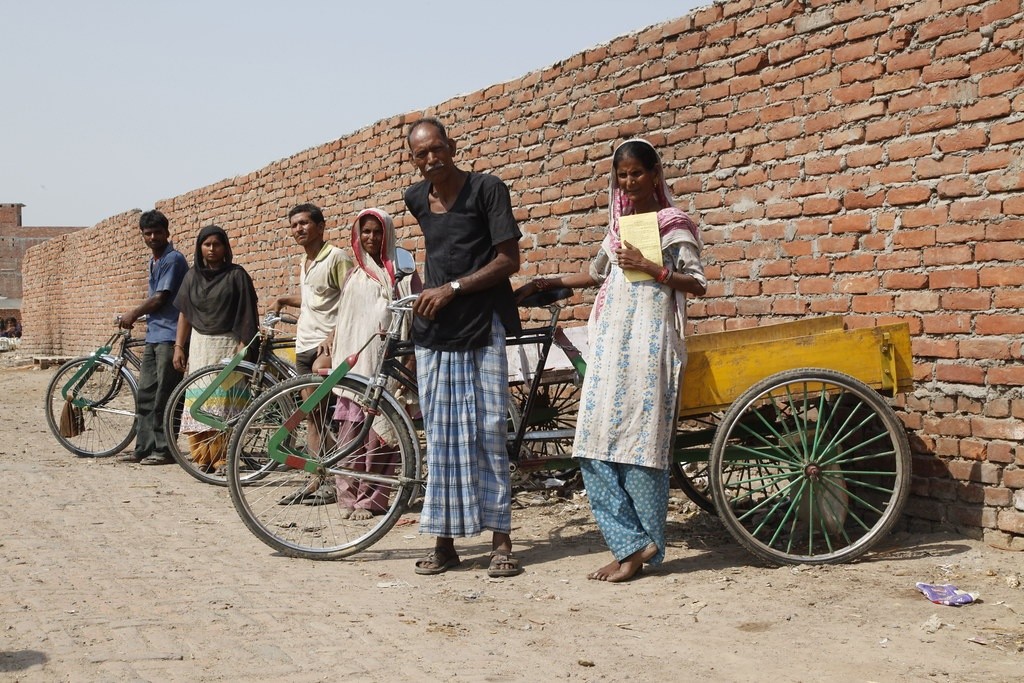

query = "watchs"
[{"left": 450, "top": 280, "right": 461, "bottom": 295}]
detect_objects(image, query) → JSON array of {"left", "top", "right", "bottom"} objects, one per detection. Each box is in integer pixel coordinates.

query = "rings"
[{"left": 329, "top": 347, "right": 332, "bottom": 349}]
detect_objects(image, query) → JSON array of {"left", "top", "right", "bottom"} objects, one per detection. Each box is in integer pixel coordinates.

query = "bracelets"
[
  {"left": 534, "top": 277, "right": 552, "bottom": 292},
  {"left": 655, "top": 267, "right": 673, "bottom": 284},
  {"left": 173, "top": 344, "right": 184, "bottom": 352}
]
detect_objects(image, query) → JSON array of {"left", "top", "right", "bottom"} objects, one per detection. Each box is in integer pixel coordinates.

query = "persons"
[
  {"left": 396, "top": 117, "right": 524, "bottom": 575},
  {"left": 117, "top": 209, "right": 189, "bottom": 465},
  {"left": 0, "top": 317, "right": 22, "bottom": 338},
  {"left": 331, "top": 208, "right": 422, "bottom": 519},
  {"left": 173, "top": 225, "right": 259, "bottom": 475},
  {"left": 516, "top": 139, "right": 707, "bottom": 581},
  {"left": 265, "top": 203, "right": 354, "bottom": 504}
]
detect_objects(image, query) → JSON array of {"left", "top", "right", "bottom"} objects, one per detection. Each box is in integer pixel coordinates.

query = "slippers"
[
  {"left": 215, "top": 467, "right": 226, "bottom": 476},
  {"left": 414, "top": 546, "right": 459, "bottom": 574},
  {"left": 140, "top": 456, "right": 168, "bottom": 465},
  {"left": 277, "top": 492, "right": 304, "bottom": 504},
  {"left": 302, "top": 481, "right": 337, "bottom": 504},
  {"left": 488, "top": 551, "right": 521, "bottom": 576},
  {"left": 117, "top": 452, "right": 142, "bottom": 463}
]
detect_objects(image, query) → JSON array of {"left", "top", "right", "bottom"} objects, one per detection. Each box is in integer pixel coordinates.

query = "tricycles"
[
  {"left": 162, "top": 292, "right": 597, "bottom": 496},
  {"left": 40, "top": 308, "right": 298, "bottom": 461},
  {"left": 232, "top": 278, "right": 924, "bottom": 562}
]
